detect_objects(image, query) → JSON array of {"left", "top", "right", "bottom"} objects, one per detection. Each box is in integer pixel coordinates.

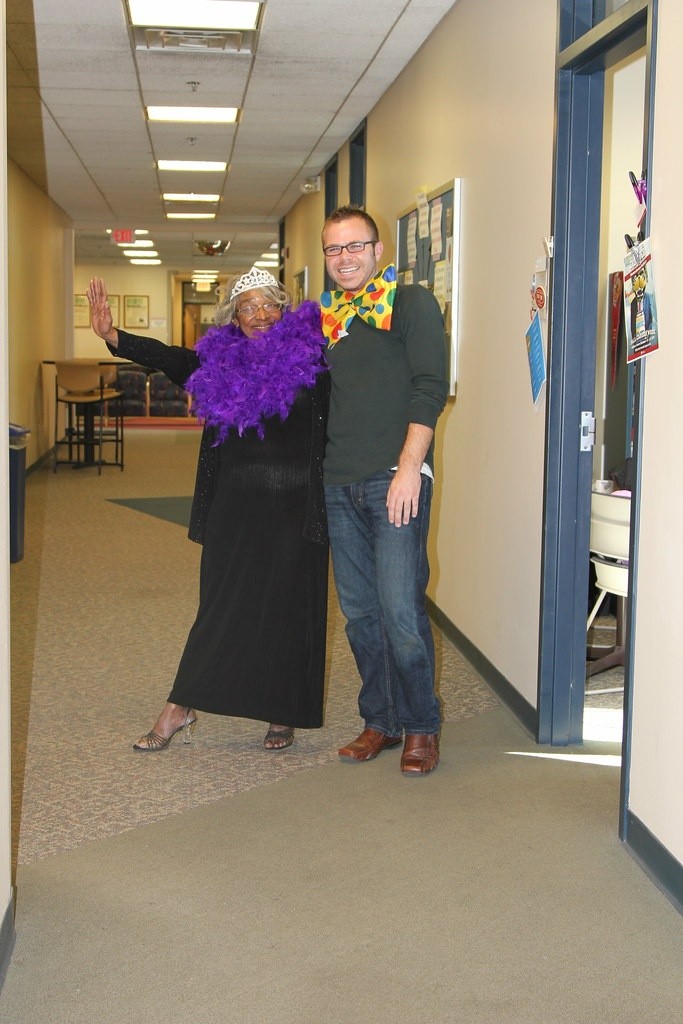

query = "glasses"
[
  {"left": 324, "top": 240, "right": 378, "bottom": 257},
  {"left": 235, "top": 301, "right": 282, "bottom": 316}
]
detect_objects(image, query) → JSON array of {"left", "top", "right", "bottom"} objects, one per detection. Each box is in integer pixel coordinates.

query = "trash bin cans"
[{"left": 9, "top": 424, "right": 31, "bottom": 564}]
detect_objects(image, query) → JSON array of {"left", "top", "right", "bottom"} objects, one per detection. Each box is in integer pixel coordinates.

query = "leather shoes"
[
  {"left": 338, "top": 729, "right": 404, "bottom": 760},
  {"left": 400, "top": 730, "right": 441, "bottom": 776}
]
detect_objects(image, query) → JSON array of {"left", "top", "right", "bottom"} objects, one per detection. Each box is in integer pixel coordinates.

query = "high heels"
[
  {"left": 263, "top": 726, "right": 296, "bottom": 748},
  {"left": 133, "top": 708, "right": 197, "bottom": 751}
]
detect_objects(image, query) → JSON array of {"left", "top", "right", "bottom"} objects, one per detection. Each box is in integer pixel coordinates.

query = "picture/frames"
[
  {"left": 73, "top": 293, "right": 91, "bottom": 328},
  {"left": 124, "top": 295, "right": 149, "bottom": 328},
  {"left": 108, "top": 294, "right": 120, "bottom": 328}
]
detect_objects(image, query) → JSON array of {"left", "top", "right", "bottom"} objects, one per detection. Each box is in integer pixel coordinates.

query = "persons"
[
  {"left": 631, "top": 265, "right": 657, "bottom": 337},
  {"left": 84, "top": 266, "right": 331, "bottom": 755},
  {"left": 314, "top": 206, "right": 448, "bottom": 778}
]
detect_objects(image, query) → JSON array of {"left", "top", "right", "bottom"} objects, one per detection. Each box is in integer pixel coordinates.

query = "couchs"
[
  {"left": 148, "top": 372, "right": 193, "bottom": 416},
  {"left": 105, "top": 369, "right": 148, "bottom": 417}
]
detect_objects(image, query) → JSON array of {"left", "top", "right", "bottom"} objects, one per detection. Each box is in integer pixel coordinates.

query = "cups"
[{"left": 592, "top": 480, "right": 615, "bottom": 494}]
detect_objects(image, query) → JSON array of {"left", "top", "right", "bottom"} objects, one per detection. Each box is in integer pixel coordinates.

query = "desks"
[{"left": 57, "top": 386, "right": 120, "bottom": 473}]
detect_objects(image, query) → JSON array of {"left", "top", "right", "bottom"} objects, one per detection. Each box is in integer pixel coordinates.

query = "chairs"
[{"left": 582, "top": 490, "right": 634, "bottom": 681}]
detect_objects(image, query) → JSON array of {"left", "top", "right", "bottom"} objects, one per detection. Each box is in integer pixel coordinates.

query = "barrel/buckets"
[{"left": 9, "top": 423, "right": 31, "bottom": 563}]
